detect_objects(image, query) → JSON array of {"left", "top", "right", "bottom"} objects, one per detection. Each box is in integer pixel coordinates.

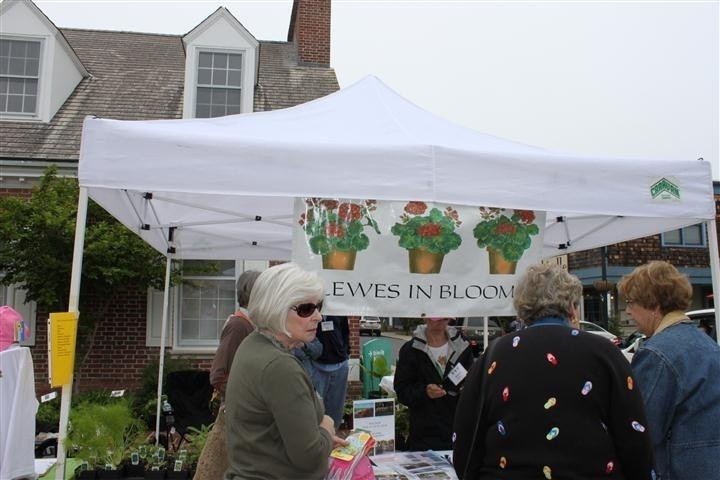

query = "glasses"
[{"left": 287, "top": 299, "right": 323, "bottom": 318}]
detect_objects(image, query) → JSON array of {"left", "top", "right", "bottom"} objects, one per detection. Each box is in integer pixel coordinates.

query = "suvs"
[{"left": 446, "top": 316, "right": 503, "bottom": 354}]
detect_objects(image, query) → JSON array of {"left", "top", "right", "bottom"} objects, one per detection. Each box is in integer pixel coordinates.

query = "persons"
[
  {"left": 214, "top": 262, "right": 346, "bottom": 480},
  {"left": 390, "top": 318, "right": 474, "bottom": 448},
  {"left": 191, "top": 271, "right": 263, "bottom": 480},
  {"left": 309, "top": 307, "right": 349, "bottom": 432},
  {"left": 291, "top": 336, "right": 324, "bottom": 377},
  {"left": 620, "top": 259, "right": 719, "bottom": 479},
  {"left": 452, "top": 267, "right": 655, "bottom": 477}
]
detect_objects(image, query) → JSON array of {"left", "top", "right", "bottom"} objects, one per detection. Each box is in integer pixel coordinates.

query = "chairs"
[{"left": 164, "top": 369, "right": 218, "bottom": 452}]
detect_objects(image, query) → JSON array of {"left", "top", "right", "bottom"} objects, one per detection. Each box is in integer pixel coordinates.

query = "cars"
[
  {"left": 621, "top": 326, "right": 644, "bottom": 348},
  {"left": 359, "top": 316, "right": 384, "bottom": 335},
  {"left": 621, "top": 307, "right": 718, "bottom": 363},
  {"left": 576, "top": 319, "right": 622, "bottom": 347}
]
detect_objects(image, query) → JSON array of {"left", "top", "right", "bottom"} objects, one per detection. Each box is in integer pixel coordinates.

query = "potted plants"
[{"left": 60, "top": 398, "right": 218, "bottom": 479}]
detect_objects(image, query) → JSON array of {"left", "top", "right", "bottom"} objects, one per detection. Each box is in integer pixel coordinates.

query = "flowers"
[
  {"left": 390, "top": 200, "right": 466, "bottom": 256},
  {"left": 472, "top": 205, "right": 540, "bottom": 261},
  {"left": 297, "top": 195, "right": 382, "bottom": 258}
]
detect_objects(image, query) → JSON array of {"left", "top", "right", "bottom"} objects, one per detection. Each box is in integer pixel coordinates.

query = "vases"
[
  {"left": 409, "top": 245, "right": 443, "bottom": 274},
  {"left": 321, "top": 244, "right": 357, "bottom": 269},
  {"left": 489, "top": 246, "right": 519, "bottom": 275}
]
